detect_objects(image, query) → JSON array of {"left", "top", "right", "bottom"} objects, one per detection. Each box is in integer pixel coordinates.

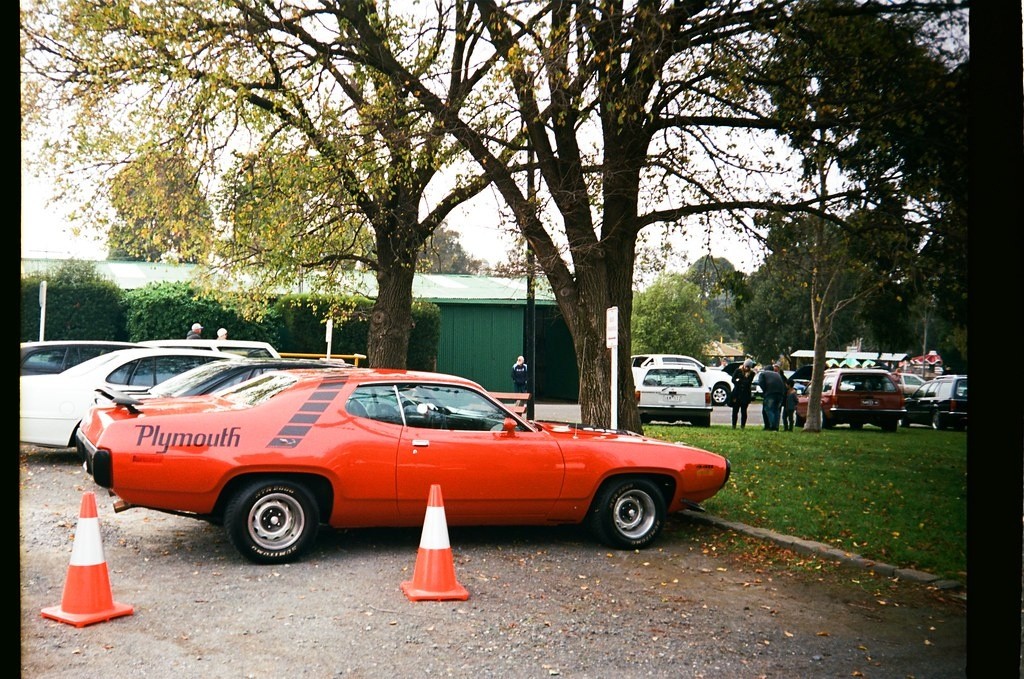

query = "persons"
[
  {"left": 186, "top": 323, "right": 203, "bottom": 339},
  {"left": 732, "top": 358, "right": 800, "bottom": 433},
  {"left": 217, "top": 328, "right": 228, "bottom": 341},
  {"left": 892, "top": 368, "right": 904, "bottom": 393},
  {"left": 511, "top": 355, "right": 528, "bottom": 408}
]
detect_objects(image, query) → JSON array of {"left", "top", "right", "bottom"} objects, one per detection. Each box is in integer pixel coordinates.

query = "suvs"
[{"left": 631, "top": 353, "right": 735, "bottom": 406}]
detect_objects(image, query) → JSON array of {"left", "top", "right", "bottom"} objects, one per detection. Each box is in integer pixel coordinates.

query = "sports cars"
[{"left": 74, "top": 367, "right": 734, "bottom": 566}]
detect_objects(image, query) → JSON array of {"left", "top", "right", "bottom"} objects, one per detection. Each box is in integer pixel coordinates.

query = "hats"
[
  {"left": 192, "top": 323, "right": 203, "bottom": 331},
  {"left": 744, "top": 358, "right": 754, "bottom": 367},
  {"left": 216, "top": 328, "right": 227, "bottom": 337}
]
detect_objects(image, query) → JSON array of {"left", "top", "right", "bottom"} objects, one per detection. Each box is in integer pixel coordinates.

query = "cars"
[
  {"left": 794, "top": 368, "right": 908, "bottom": 433},
  {"left": 722, "top": 361, "right": 927, "bottom": 408},
  {"left": 635, "top": 362, "right": 713, "bottom": 427},
  {"left": 19, "top": 338, "right": 489, "bottom": 465},
  {"left": 898, "top": 374, "right": 969, "bottom": 430}
]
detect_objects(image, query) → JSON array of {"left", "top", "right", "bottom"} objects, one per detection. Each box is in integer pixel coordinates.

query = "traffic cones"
[
  {"left": 39, "top": 491, "right": 134, "bottom": 630},
  {"left": 401, "top": 484, "right": 469, "bottom": 602}
]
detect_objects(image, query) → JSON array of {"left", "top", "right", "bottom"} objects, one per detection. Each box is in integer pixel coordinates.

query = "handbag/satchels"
[{"left": 727, "top": 382, "right": 746, "bottom": 407}]
